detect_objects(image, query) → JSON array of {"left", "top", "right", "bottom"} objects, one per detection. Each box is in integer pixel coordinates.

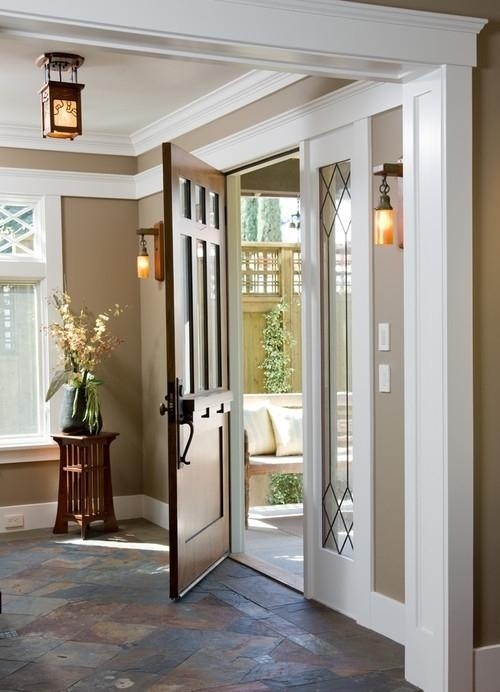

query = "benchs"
[{"left": 242, "top": 387, "right": 352, "bottom": 534}]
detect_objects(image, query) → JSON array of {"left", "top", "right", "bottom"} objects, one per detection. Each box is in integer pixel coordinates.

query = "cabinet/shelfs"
[{"left": 50, "top": 430, "right": 124, "bottom": 539}]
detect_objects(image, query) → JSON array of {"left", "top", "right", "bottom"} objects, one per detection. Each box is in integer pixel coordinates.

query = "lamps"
[
  {"left": 35, "top": 50, "right": 88, "bottom": 145},
  {"left": 371, "top": 156, "right": 409, "bottom": 251},
  {"left": 134, "top": 218, "right": 169, "bottom": 284}
]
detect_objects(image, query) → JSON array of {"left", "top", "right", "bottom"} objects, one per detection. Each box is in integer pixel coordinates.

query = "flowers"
[{"left": 41, "top": 280, "right": 131, "bottom": 435}]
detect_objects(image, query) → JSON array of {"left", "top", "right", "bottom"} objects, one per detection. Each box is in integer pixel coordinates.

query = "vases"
[{"left": 61, "top": 385, "right": 104, "bottom": 435}]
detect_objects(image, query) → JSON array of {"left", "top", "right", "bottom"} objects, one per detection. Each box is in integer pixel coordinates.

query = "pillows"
[{"left": 242, "top": 399, "right": 304, "bottom": 459}]
